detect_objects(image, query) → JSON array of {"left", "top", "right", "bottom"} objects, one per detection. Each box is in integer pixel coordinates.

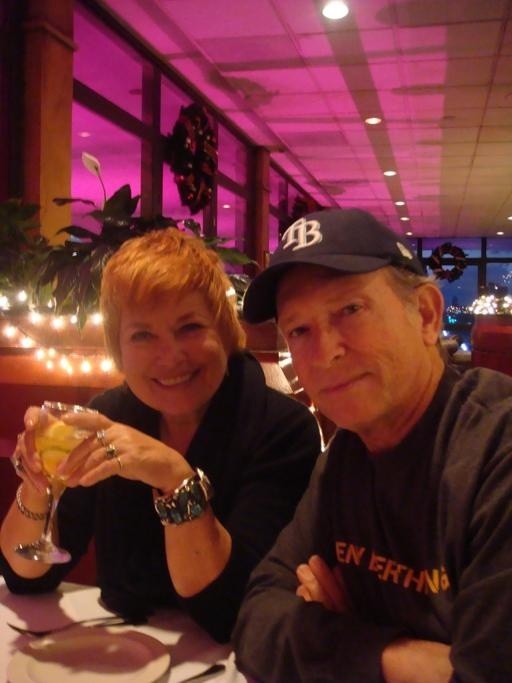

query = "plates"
[{"left": 4, "top": 625, "right": 174, "bottom": 683}]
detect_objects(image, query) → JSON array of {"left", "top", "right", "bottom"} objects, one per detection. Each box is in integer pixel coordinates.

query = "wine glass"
[{"left": 14, "top": 398, "right": 99, "bottom": 565}]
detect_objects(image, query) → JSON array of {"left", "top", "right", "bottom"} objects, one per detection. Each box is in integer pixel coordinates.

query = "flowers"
[{"left": 0, "top": 153, "right": 264, "bottom": 337}]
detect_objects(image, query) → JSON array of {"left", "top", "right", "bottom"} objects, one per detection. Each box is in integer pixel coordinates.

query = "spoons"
[{"left": 3, "top": 612, "right": 149, "bottom": 641}]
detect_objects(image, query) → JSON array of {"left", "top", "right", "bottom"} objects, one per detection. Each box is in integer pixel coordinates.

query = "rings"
[
  {"left": 115, "top": 453, "right": 124, "bottom": 474},
  {"left": 106, "top": 442, "right": 118, "bottom": 457},
  {"left": 95, "top": 425, "right": 109, "bottom": 446},
  {"left": 15, "top": 430, "right": 25, "bottom": 440},
  {"left": 13, "top": 454, "right": 24, "bottom": 471}
]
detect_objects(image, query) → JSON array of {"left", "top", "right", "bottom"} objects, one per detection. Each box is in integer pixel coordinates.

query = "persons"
[
  {"left": 1, "top": 227, "right": 322, "bottom": 646},
  {"left": 231, "top": 201, "right": 512, "bottom": 683}
]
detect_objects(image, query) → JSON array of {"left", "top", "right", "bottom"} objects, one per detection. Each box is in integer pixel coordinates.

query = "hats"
[{"left": 241, "top": 207, "right": 428, "bottom": 325}]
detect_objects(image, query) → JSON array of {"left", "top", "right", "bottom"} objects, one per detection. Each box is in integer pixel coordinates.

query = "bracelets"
[
  {"left": 151, "top": 467, "right": 213, "bottom": 527},
  {"left": 15, "top": 480, "right": 53, "bottom": 522}
]
